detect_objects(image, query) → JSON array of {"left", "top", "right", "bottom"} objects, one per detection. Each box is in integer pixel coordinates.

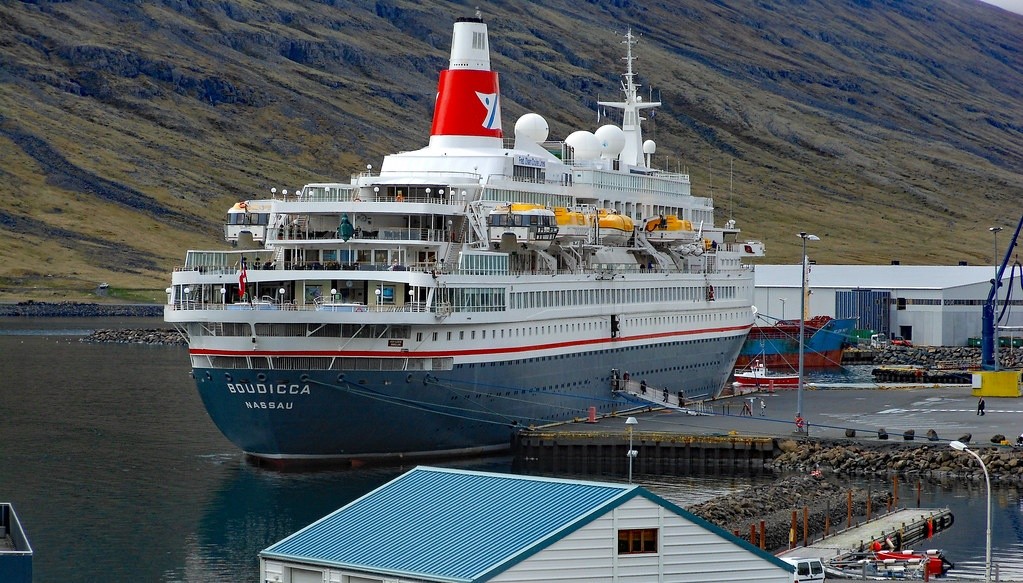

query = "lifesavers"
[
  {"left": 795, "top": 417, "right": 804, "bottom": 428},
  {"left": 395, "top": 196, "right": 403, "bottom": 203},
  {"left": 354, "top": 198, "right": 362, "bottom": 203}
]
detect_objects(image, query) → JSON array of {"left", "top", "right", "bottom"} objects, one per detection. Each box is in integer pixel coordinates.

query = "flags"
[{"left": 238, "top": 269, "right": 246, "bottom": 297}]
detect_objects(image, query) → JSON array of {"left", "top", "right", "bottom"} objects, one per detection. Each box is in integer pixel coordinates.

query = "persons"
[
  {"left": 760, "top": 399, "right": 766, "bottom": 416},
  {"left": 265, "top": 260, "right": 272, "bottom": 266},
  {"left": 243, "top": 257, "right": 247, "bottom": 268},
  {"left": 640, "top": 263, "right": 645, "bottom": 273},
  {"left": 254, "top": 258, "right": 260, "bottom": 270},
  {"left": 624, "top": 371, "right": 629, "bottom": 380},
  {"left": 979, "top": 397, "right": 985, "bottom": 416},
  {"left": 678, "top": 390, "right": 684, "bottom": 408},
  {"left": 381, "top": 259, "right": 397, "bottom": 271},
  {"left": 663, "top": 387, "right": 669, "bottom": 402},
  {"left": 641, "top": 379, "right": 647, "bottom": 395},
  {"left": 648, "top": 260, "right": 652, "bottom": 273}
]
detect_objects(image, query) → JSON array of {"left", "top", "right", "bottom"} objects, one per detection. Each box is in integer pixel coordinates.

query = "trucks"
[{"left": 849, "top": 328, "right": 887, "bottom": 350}]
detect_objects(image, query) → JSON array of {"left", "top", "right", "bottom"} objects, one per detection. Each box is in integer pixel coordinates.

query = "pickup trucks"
[{"left": 891, "top": 336, "right": 908, "bottom": 347}]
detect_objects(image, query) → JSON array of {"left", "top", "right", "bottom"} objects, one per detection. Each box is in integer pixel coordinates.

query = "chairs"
[
  {"left": 284, "top": 299, "right": 299, "bottom": 311},
  {"left": 292, "top": 261, "right": 358, "bottom": 270},
  {"left": 303, "top": 229, "right": 419, "bottom": 240}
]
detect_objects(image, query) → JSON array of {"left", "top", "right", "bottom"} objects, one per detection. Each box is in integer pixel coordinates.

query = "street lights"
[
  {"left": 793, "top": 231, "right": 822, "bottom": 432},
  {"left": 949, "top": 440, "right": 992, "bottom": 583},
  {"left": 988, "top": 226, "right": 1004, "bottom": 372},
  {"left": 778, "top": 298, "right": 785, "bottom": 320},
  {"left": 623, "top": 417, "right": 639, "bottom": 484}
]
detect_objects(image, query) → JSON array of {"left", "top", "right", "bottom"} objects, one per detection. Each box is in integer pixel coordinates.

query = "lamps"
[
  {"left": 409, "top": 289, "right": 414, "bottom": 296},
  {"left": 271, "top": 165, "right": 479, "bottom": 195},
  {"left": 166, "top": 287, "right": 172, "bottom": 294},
  {"left": 375, "top": 289, "right": 380, "bottom": 295},
  {"left": 279, "top": 288, "right": 285, "bottom": 294},
  {"left": 184, "top": 287, "right": 190, "bottom": 294},
  {"left": 221, "top": 288, "right": 226, "bottom": 294},
  {"left": 331, "top": 288, "right": 337, "bottom": 294}
]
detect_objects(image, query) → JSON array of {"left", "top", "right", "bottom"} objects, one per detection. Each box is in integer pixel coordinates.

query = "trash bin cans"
[{"left": 968, "top": 337, "right": 1023, "bottom": 348}]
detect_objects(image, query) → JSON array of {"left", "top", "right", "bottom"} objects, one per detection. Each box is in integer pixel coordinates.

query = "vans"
[{"left": 778, "top": 556, "right": 825, "bottom": 583}]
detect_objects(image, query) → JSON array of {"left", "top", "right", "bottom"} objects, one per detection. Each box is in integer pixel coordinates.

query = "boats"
[
  {"left": 645, "top": 215, "right": 696, "bottom": 244},
  {"left": 488, "top": 201, "right": 559, "bottom": 251},
  {"left": 733, "top": 314, "right": 860, "bottom": 369},
  {"left": 820, "top": 540, "right": 956, "bottom": 583},
  {"left": 163, "top": 9, "right": 767, "bottom": 474},
  {"left": 732, "top": 338, "right": 799, "bottom": 388},
  {"left": 587, "top": 208, "right": 635, "bottom": 244},
  {"left": 553, "top": 206, "right": 591, "bottom": 240}
]
